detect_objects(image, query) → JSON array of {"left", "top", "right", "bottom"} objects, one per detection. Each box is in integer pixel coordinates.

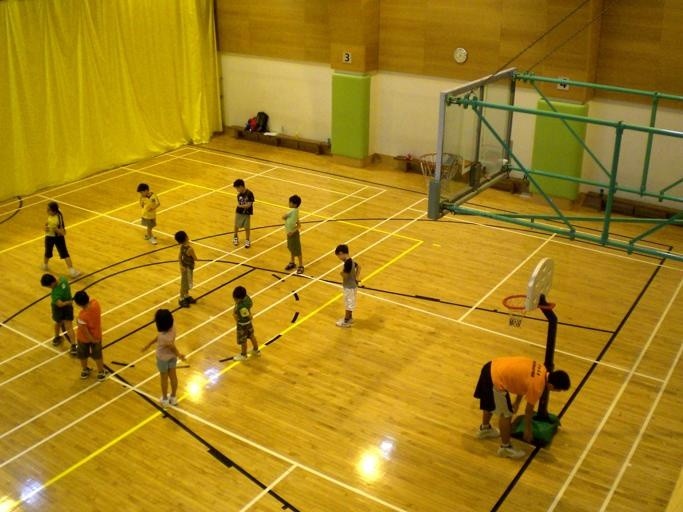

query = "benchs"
[
  {"left": 235, "top": 126, "right": 328, "bottom": 155},
  {"left": 393, "top": 156, "right": 527, "bottom": 195},
  {"left": 584, "top": 190, "right": 680, "bottom": 220}
]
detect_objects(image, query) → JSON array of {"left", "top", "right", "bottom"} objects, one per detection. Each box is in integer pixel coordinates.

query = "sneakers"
[
  {"left": 79, "top": 364, "right": 105, "bottom": 381},
  {"left": 233, "top": 238, "right": 252, "bottom": 249},
  {"left": 179, "top": 296, "right": 196, "bottom": 308},
  {"left": 160, "top": 393, "right": 177, "bottom": 406},
  {"left": 336, "top": 319, "right": 353, "bottom": 327},
  {"left": 497, "top": 443, "right": 526, "bottom": 457},
  {"left": 233, "top": 349, "right": 262, "bottom": 360},
  {"left": 145, "top": 235, "right": 157, "bottom": 245},
  {"left": 51, "top": 335, "right": 79, "bottom": 356},
  {"left": 71, "top": 272, "right": 80, "bottom": 278},
  {"left": 285, "top": 263, "right": 305, "bottom": 275},
  {"left": 474, "top": 424, "right": 500, "bottom": 438}
]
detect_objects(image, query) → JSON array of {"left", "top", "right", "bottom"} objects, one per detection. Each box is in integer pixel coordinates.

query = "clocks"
[{"left": 452, "top": 46, "right": 467, "bottom": 64}]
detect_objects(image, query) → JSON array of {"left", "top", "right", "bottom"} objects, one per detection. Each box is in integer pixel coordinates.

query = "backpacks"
[{"left": 252, "top": 112, "right": 267, "bottom": 131}]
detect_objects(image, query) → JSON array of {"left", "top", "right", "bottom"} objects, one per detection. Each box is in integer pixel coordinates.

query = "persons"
[
  {"left": 231, "top": 177, "right": 254, "bottom": 248},
  {"left": 39, "top": 272, "right": 78, "bottom": 359},
  {"left": 71, "top": 289, "right": 107, "bottom": 382},
  {"left": 135, "top": 182, "right": 161, "bottom": 246},
  {"left": 281, "top": 194, "right": 308, "bottom": 274},
  {"left": 39, "top": 200, "right": 78, "bottom": 278},
  {"left": 140, "top": 307, "right": 186, "bottom": 408},
  {"left": 469, "top": 354, "right": 572, "bottom": 459},
  {"left": 172, "top": 230, "right": 197, "bottom": 308},
  {"left": 333, "top": 242, "right": 361, "bottom": 328},
  {"left": 231, "top": 285, "right": 260, "bottom": 361}
]
